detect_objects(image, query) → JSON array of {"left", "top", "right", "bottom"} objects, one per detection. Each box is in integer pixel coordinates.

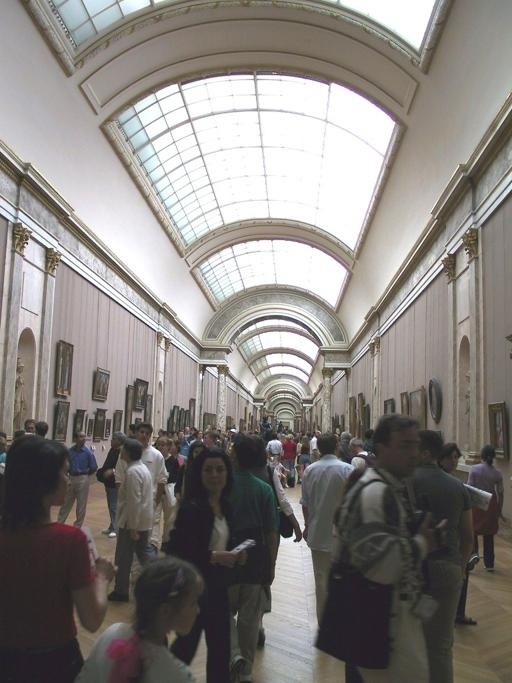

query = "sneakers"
[
  {"left": 456, "top": 616, "right": 476, "bottom": 625},
  {"left": 107, "top": 591, "right": 130, "bottom": 601},
  {"left": 101, "top": 527, "right": 111, "bottom": 533},
  {"left": 108, "top": 531, "right": 117, "bottom": 537},
  {"left": 227, "top": 625, "right": 266, "bottom": 682},
  {"left": 482, "top": 564, "right": 494, "bottom": 573},
  {"left": 466, "top": 552, "right": 480, "bottom": 570}
]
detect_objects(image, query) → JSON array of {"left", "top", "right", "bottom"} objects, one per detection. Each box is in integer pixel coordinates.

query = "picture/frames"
[
  {"left": 488, "top": 402, "right": 509, "bottom": 462},
  {"left": 53, "top": 340, "right": 152, "bottom": 443},
  {"left": 349, "top": 393, "right": 370, "bottom": 437},
  {"left": 384, "top": 378, "right": 442, "bottom": 431},
  {"left": 172, "top": 398, "right": 195, "bottom": 432}
]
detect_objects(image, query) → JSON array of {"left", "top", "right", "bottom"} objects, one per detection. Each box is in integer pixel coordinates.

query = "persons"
[{"left": 1, "top": 410, "right": 511, "bottom": 683}]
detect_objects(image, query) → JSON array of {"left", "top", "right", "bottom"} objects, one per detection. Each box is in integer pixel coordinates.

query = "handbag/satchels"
[
  {"left": 311, "top": 560, "right": 393, "bottom": 671},
  {"left": 278, "top": 510, "right": 294, "bottom": 538}
]
